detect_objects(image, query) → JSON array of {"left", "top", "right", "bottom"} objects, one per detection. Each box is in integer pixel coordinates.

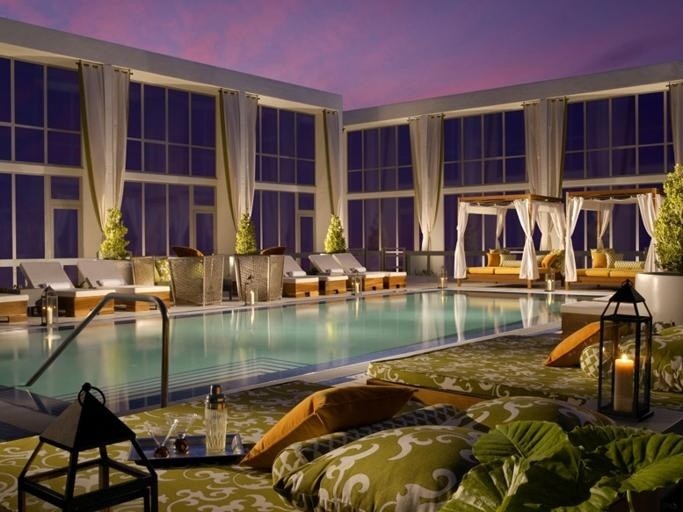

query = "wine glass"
[{"left": 144, "top": 412, "right": 197, "bottom": 457}]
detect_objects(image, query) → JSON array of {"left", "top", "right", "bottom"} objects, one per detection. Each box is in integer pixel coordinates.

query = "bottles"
[{"left": 204, "top": 383, "right": 227, "bottom": 452}]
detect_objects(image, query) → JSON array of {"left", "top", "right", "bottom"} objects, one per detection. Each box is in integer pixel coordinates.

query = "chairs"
[
  {"left": 282, "top": 252, "right": 407, "bottom": 298},
  {"left": 18, "top": 260, "right": 172, "bottom": 319}
]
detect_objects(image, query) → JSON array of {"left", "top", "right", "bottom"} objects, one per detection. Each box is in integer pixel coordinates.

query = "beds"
[
  {"left": 457, "top": 249, "right": 566, "bottom": 289},
  {"left": 565, "top": 251, "right": 648, "bottom": 291}
]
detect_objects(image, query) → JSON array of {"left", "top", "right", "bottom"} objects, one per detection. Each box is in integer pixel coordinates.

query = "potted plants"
[{"left": 634, "top": 162, "right": 682, "bottom": 331}]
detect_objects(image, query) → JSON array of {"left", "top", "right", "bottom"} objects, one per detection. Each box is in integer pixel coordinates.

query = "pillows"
[
  {"left": 541, "top": 253, "right": 558, "bottom": 268},
  {"left": 486, "top": 249, "right": 516, "bottom": 268},
  {"left": 545, "top": 318, "right": 683, "bottom": 392},
  {"left": 590, "top": 248, "right": 624, "bottom": 269}
]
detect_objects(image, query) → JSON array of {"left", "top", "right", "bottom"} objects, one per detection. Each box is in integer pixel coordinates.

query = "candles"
[{"left": 612, "top": 351, "right": 635, "bottom": 411}]
